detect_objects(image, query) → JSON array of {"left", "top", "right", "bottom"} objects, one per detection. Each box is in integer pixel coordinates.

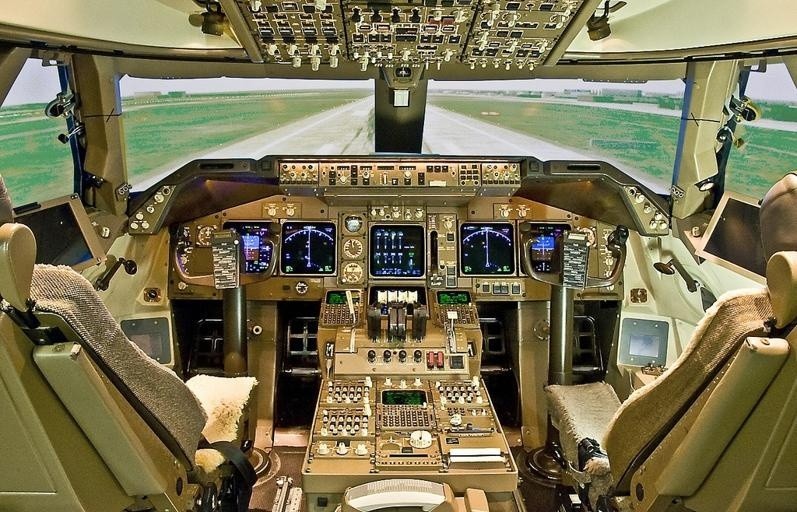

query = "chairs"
[
  {"left": 0, "top": 172, "right": 261, "bottom": 511},
  {"left": 544, "top": 170, "right": 797, "bottom": 510}
]
